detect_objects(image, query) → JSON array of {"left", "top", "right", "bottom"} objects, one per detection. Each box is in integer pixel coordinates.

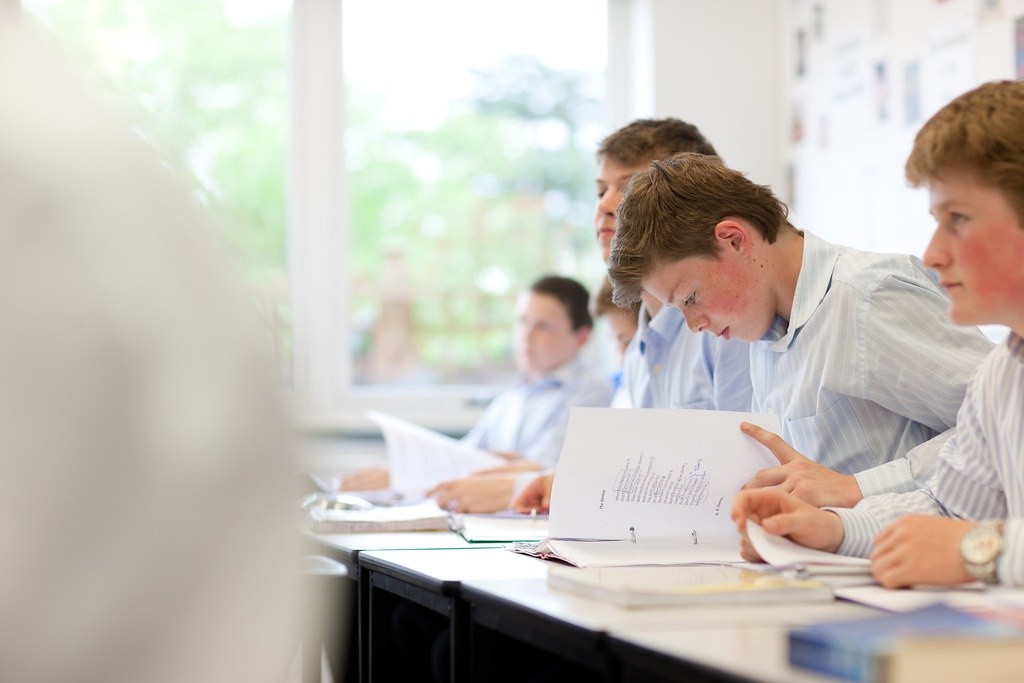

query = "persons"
[
  {"left": 337, "top": 115, "right": 993, "bottom": 515},
  {"left": 731, "top": 78, "right": 1024, "bottom": 590},
  {"left": 2, "top": 0, "right": 311, "bottom": 683}
]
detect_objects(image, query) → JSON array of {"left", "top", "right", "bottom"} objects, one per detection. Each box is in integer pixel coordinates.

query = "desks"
[{"left": 306, "top": 531, "right": 1024, "bottom": 683}]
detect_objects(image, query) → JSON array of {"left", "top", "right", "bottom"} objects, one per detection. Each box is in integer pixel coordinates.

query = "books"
[{"left": 786, "top": 600, "right": 1024, "bottom": 683}]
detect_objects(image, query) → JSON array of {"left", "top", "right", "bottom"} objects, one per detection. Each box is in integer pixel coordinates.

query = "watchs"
[{"left": 959, "top": 517, "right": 1006, "bottom": 584}]
